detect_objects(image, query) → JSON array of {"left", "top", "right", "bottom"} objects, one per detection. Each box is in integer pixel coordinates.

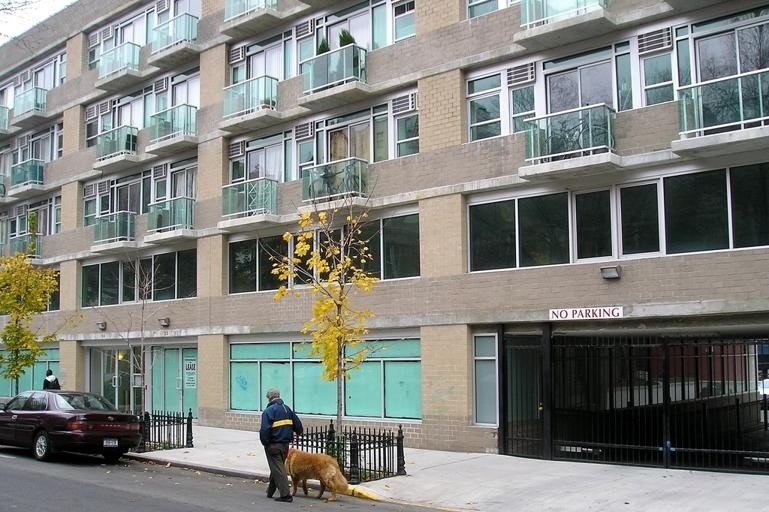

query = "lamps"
[
  {"left": 598, "top": 264, "right": 623, "bottom": 281},
  {"left": 95, "top": 320, "right": 107, "bottom": 331},
  {"left": 157, "top": 316, "right": 171, "bottom": 327}
]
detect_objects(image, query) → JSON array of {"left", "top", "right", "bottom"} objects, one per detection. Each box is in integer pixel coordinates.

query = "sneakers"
[
  {"left": 266, "top": 488, "right": 273, "bottom": 498},
  {"left": 275, "top": 496, "right": 293, "bottom": 502}
]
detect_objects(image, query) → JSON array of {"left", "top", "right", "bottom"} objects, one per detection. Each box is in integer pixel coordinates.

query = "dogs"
[{"left": 283, "top": 448, "right": 349, "bottom": 501}]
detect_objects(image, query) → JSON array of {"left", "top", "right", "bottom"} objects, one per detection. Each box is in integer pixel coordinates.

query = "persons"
[
  {"left": 259, "top": 389, "right": 303, "bottom": 502},
  {"left": 43, "top": 369, "right": 60, "bottom": 389}
]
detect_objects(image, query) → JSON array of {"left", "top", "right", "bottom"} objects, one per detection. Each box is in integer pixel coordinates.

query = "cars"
[{"left": 0, "top": 388, "right": 141, "bottom": 460}]
